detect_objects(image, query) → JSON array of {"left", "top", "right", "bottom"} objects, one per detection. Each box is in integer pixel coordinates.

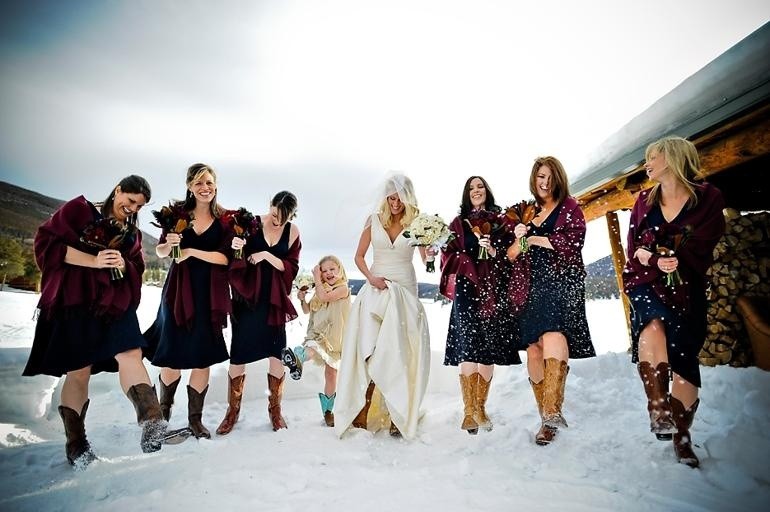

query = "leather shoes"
[{"left": 389, "top": 419, "right": 402, "bottom": 436}]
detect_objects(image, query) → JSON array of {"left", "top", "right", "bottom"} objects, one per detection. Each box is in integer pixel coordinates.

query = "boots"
[
  {"left": 668, "top": 391, "right": 701, "bottom": 469},
  {"left": 127, "top": 375, "right": 192, "bottom": 452},
  {"left": 637, "top": 360, "right": 678, "bottom": 441},
  {"left": 319, "top": 392, "right": 336, "bottom": 427},
  {"left": 158, "top": 373, "right": 181, "bottom": 423},
  {"left": 281, "top": 345, "right": 306, "bottom": 380},
  {"left": 267, "top": 372, "right": 288, "bottom": 432},
  {"left": 458, "top": 372, "right": 494, "bottom": 435},
  {"left": 215, "top": 371, "right": 246, "bottom": 436},
  {"left": 528, "top": 357, "right": 572, "bottom": 445},
  {"left": 351, "top": 380, "right": 375, "bottom": 430},
  {"left": 58, "top": 399, "right": 102, "bottom": 472},
  {"left": 186, "top": 384, "right": 211, "bottom": 439}
]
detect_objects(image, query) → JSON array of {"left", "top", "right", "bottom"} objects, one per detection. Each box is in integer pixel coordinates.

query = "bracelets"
[{"left": 539, "top": 235, "right": 542, "bottom": 249}]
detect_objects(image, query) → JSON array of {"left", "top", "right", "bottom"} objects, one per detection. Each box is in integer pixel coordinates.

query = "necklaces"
[{"left": 540, "top": 206, "right": 554, "bottom": 211}]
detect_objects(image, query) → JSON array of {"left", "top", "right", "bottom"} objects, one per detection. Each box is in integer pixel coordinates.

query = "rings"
[{"left": 665, "top": 265, "right": 668, "bottom": 270}]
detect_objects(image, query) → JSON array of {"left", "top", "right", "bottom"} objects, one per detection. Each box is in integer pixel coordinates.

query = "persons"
[
  {"left": 141, "top": 164, "right": 234, "bottom": 441},
  {"left": 502, "top": 157, "right": 597, "bottom": 445},
  {"left": 440, "top": 176, "right": 508, "bottom": 433},
  {"left": 21, "top": 176, "right": 194, "bottom": 471},
  {"left": 214, "top": 192, "right": 302, "bottom": 436},
  {"left": 624, "top": 136, "right": 727, "bottom": 467},
  {"left": 340, "top": 172, "right": 438, "bottom": 443},
  {"left": 285, "top": 255, "right": 351, "bottom": 426}
]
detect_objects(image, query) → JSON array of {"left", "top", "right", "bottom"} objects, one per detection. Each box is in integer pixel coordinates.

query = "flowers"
[
  {"left": 223, "top": 205, "right": 266, "bottom": 263},
  {"left": 400, "top": 212, "right": 465, "bottom": 275},
  {"left": 78, "top": 211, "right": 132, "bottom": 283},
  {"left": 457, "top": 202, "right": 511, "bottom": 259},
  {"left": 629, "top": 218, "right": 699, "bottom": 289},
  {"left": 289, "top": 270, "right": 318, "bottom": 308},
  {"left": 145, "top": 198, "right": 201, "bottom": 259},
  {"left": 501, "top": 195, "right": 549, "bottom": 257}
]
